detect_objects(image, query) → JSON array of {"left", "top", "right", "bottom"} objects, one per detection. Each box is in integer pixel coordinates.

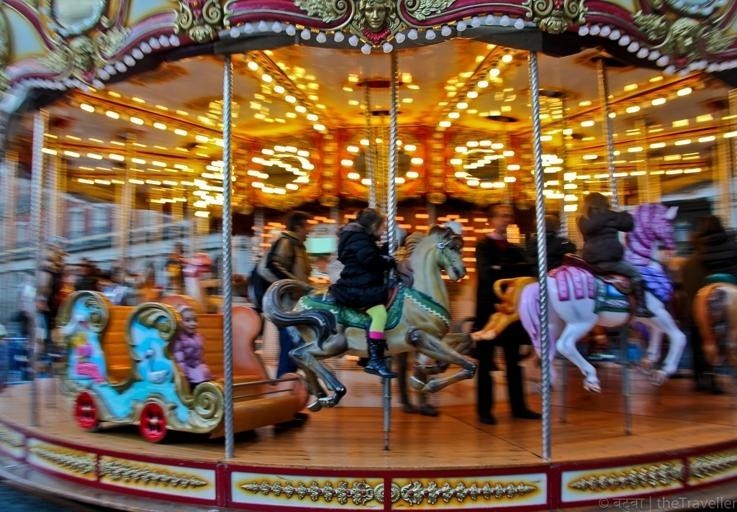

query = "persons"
[
  {"left": 534, "top": 215, "right": 574, "bottom": 274},
  {"left": 15, "top": 241, "right": 195, "bottom": 372},
  {"left": 673, "top": 197, "right": 737, "bottom": 397},
  {"left": 267, "top": 210, "right": 312, "bottom": 379},
  {"left": 327, "top": 206, "right": 400, "bottom": 379},
  {"left": 471, "top": 201, "right": 543, "bottom": 426},
  {"left": 576, "top": 191, "right": 655, "bottom": 318}
]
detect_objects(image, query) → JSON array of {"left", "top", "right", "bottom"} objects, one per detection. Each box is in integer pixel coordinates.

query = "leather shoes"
[
  {"left": 475, "top": 409, "right": 497, "bottom": 427},
  {"left": 271, "top": 412, "right": 311, "bottom": 432},
  {"left": 509, "top": 406, "right": 543, "bottom": 420}
]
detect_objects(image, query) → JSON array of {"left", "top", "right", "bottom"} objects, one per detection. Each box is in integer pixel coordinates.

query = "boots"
[
  {"left": 363, "top": 335, "right": 401, "bottom": 380},
  {"left": 632, "top": 279, "right": 658, "bottom": 321}
]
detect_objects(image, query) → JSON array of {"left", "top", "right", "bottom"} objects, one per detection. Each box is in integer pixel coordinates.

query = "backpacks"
[{"left": 247, "top": 253, "right": 278, "bottom": 313}]
